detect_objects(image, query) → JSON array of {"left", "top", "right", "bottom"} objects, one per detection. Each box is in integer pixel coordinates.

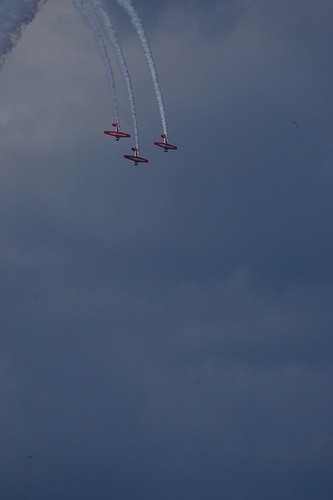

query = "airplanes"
[
  {"left": 123, "top": 147, "right": 149, "bottom": 166},
  {"left": 104, "top": 123, "right": 131, "bottom": 141},
  {"left": 153, "top": 134, "right": 178, "bottom": 152}
]
